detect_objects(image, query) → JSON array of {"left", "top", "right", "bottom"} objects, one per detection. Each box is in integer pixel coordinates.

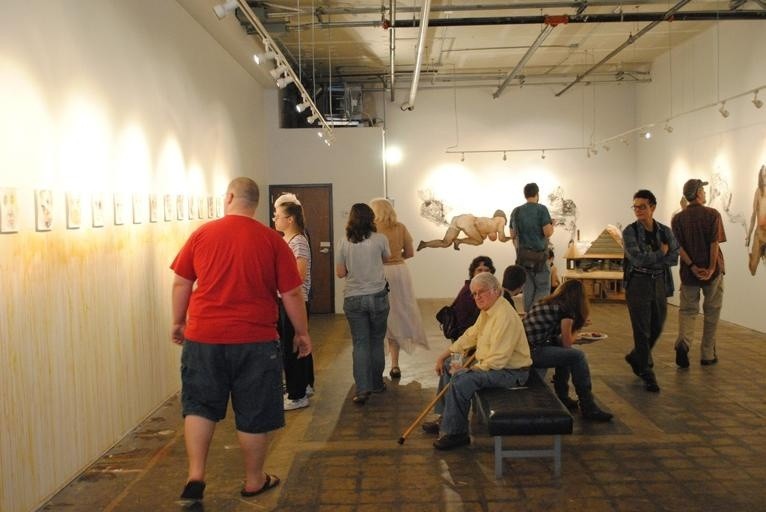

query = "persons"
[
  {"left": 547, "top": 247, "right": 561, "bottom": 293},
  {"left": 745, "top": 163, "right": 766, "bottom": 275},
  {"left": 508, "top": 182, "right": 555, "bottom": 312},
  {"left": 521, "top": 278, "right": 614, "bottom": 421},
  {"left": 169, "top": 176, "right": 313, "bottom": 500},
  {"left": 416, "top": 208, "right": 512, "bottom": 251},
  {"left": 337, "top": 202, "right": 393, "bottom": 405},
  {"left": 623, "top": 189, "right": 679, "bottom": 393},
  {"left": 454, "top": 255, "right": 515, "bottom": 333},
  {"left": 423, "top": 271, "right": 533, "bottom": 449},
  {"left": 274, "top": 190, "right": 314, "bottom": 397},
  {"left": 372, "top": 198, "right": 430, "bottom": 379},
  {"left": 500, "top": 265, "right": 526, "bottom": 313},
  {"left": 672, "top": 178, "right": 730, "bottom": 367},
  {"left": 271, "top": 202, "right": 312, "bottom": 412}
]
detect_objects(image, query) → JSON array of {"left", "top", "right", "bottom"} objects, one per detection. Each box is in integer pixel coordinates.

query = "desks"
[{"left": 471, "top": 365, "right": 573, "bottom": 480}]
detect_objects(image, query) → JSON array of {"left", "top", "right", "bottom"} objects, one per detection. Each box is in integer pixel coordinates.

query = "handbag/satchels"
[
  {"left": 514, "top": 248, "right": 548, "bottom": 271},
  {"left": 437, "top": 307, "right": 476, "bottom": 338}
]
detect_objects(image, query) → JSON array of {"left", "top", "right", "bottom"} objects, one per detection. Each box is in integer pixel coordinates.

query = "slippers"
[
  {"left": 181, "top": 481, "right": 205, "bottom": 500},
  {"left": 240, "top": 474, "right": 278, "bottom": 498},
  {"left": 353, "top": 394, "right": 369, "bottom": 403}
]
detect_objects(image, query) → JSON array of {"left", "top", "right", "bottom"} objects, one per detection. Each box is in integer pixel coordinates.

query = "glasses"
[
  {"left": 631, "top": 204, "right": 652, "bottom": 209},
  {"left": 272, "top": 215, "right": 288, "bottom": 222}
]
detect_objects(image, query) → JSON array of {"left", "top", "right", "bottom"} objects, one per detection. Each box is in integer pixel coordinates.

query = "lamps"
[
  {"left": 592, "top": 1, "right": 764, "bottom": 155},
  {"left": 211, "top": 0, "right": 335, "bottom": 149},
  {"left": 445, "top": 145, "right": 592, "bottom": 161}
]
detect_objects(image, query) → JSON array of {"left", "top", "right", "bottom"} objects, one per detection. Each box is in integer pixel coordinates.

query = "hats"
[{"left": 683, "top": 180, "right": 708, "bottom": 198}]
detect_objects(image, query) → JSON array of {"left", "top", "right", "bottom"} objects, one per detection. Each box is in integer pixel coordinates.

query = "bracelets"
[{"left": 689, "top": 262, "right": 695, "bottom": 268}]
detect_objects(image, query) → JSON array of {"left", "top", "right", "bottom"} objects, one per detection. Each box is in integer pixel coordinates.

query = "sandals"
[{"left": 390, "top": 367, "right": 400, "bottom": 377}]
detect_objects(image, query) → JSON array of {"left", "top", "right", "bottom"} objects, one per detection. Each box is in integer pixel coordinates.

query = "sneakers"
[
  {"left": 282, "top": 384, "right": 314, "bottom": 411},
  {"left": 625, "top": 354, "right": 660, "bottom": 392},
  {"left": 423, "top": 416, "right": 442, "bottom": 432},
  {"left": 433, "top": 432, "right": 470, "bottom": 449},
  {"left": 676, "top": 342, "right": 689, "bottom": 367}
]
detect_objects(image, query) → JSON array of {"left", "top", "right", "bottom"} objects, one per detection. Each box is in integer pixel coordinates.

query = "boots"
[
  {"left": 577, "top": 386, "right": 613, "bottom": 420},
  {"left": 551, "top": 374, "right": 578, "bottom": 409}
]
